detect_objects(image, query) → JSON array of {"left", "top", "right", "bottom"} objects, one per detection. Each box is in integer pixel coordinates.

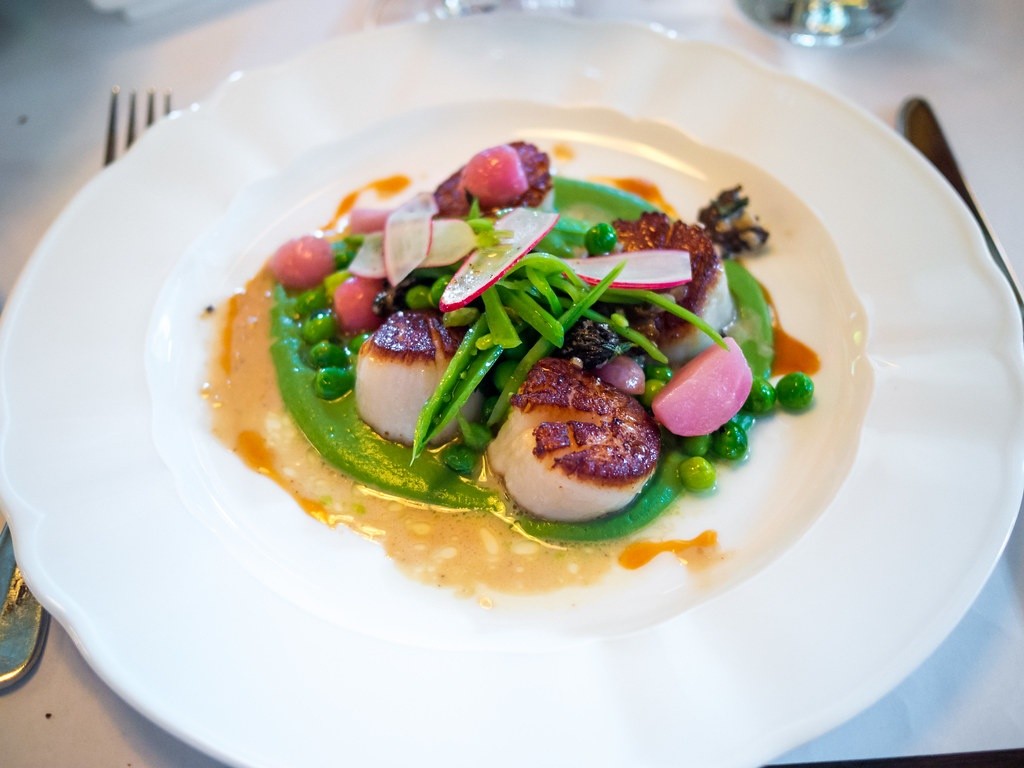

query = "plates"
[{"left": 0, "top": 13, "right": 1024, "bottom": 768}]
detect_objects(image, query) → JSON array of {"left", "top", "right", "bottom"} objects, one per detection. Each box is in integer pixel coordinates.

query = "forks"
[{"left": 0, "top": 88, "right": 169, "bottom": 690}]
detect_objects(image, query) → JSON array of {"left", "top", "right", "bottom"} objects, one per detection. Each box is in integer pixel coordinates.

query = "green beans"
[{"left": 297, "top": 222, "right": 813, "bottom": 491}]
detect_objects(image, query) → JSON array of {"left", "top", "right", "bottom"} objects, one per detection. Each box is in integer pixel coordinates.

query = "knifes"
[{"left": 899, "top": 98, "right": 1024, "bottom": 344}]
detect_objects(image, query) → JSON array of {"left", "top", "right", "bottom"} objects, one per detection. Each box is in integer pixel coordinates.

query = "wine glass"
[{"left": 744, "top": 0, "right": 901, "bottom": 47}]
